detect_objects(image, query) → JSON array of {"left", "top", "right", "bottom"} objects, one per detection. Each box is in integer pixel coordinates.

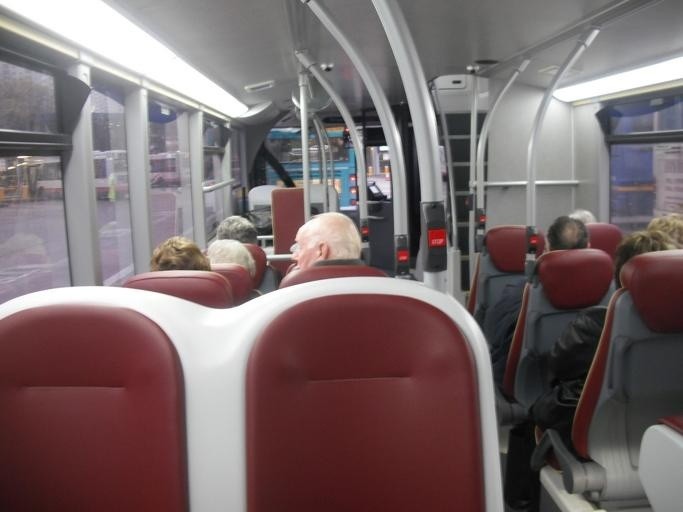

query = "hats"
[{"left": 149, "top": 141, "right": 179, "bottom": 154}]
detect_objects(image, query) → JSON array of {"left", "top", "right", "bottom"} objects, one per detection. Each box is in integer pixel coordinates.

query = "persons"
[
  {"left": 649, "top": 213, "right": 682, "bottom": 251},
  {"left": 567, "top": 211, "right": 597, "bottom": 224},
  {"left": 503, "top": 230, "right": 678, "bottom": 510},
  {"left": 148, "top": 206, "right": 366, "bottom": 273},
  {"left": 488, "top": 216, "right": 590, "bottom": 384}
]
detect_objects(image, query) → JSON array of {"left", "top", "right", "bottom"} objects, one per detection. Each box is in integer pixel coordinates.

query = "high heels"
[{"left": 384, "top": 166, "right": 390, "bottom": 182}]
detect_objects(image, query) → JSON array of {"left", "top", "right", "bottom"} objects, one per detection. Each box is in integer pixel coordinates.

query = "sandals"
[
  {"left": 149, "top": 150, "right": 210, "bottom": 189},
  {"left": 0, "top": 156, "right": 32, "bottom": 202},
  {"left": 212, "top": 126, "right": 358, "bottom": 215},
  {"left": 608, "top": 92, "right": 683, "bottom": 237},
  {"left": 27, "top": 149, "right": 129, "bottom": 202}
]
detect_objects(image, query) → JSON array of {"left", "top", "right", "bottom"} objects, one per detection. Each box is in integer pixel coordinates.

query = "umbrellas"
[{"left": 505, "top": 495, "right": 537, "bottom": 511}]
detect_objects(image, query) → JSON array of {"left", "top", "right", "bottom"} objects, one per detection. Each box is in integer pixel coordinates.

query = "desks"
[
  {"left": 107, "top": 120, "right": 122, "bottom": 150},
  {"left": 366, "top": 166, "right": 374, "bottom": 177}
]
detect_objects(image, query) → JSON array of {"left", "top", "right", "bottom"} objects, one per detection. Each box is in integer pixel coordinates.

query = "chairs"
[{"left": 0, "top": 223, "right": 683, "bottom": 512}]
